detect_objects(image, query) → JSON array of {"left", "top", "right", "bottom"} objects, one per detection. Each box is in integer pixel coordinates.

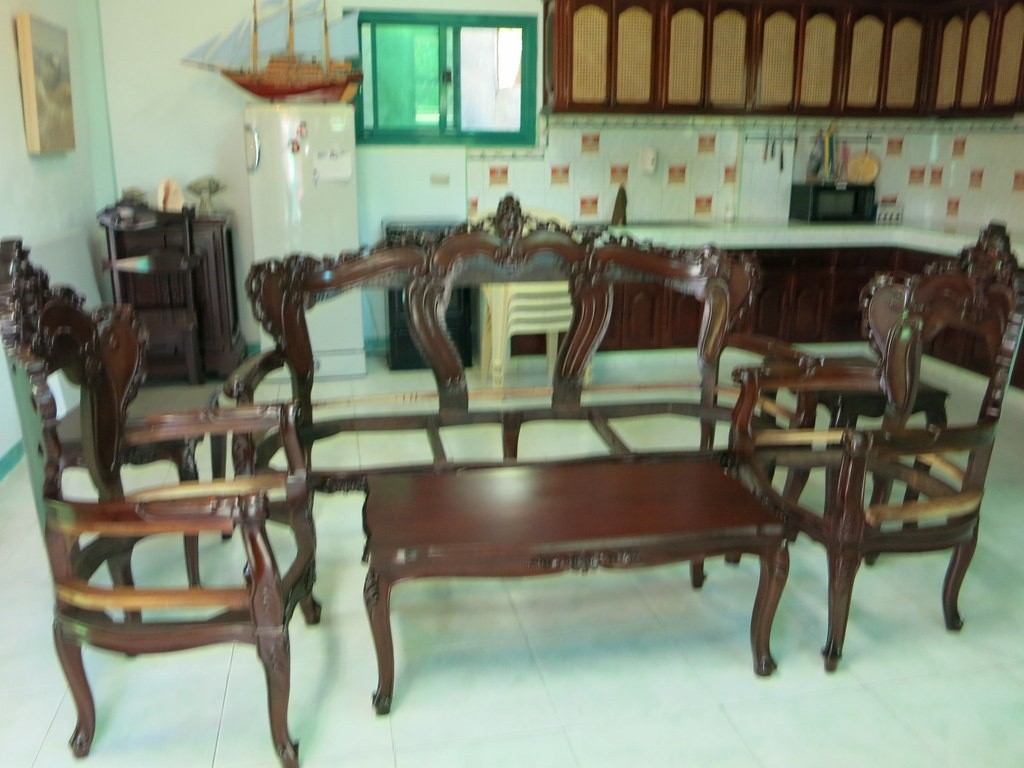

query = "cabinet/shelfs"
[
  {"left": 383, "top": 222, "right": 472, "bottom": 369},
  {"left": 511, "top": 247, "right": 1024, "bottom": 390},
  {"left": 102, "top": 213, "right": 247, "bottom": 381}
]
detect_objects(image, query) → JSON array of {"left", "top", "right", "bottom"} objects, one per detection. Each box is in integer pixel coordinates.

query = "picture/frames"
[{"left": 15, "top": 9, "right": 75, "bottom": 155}]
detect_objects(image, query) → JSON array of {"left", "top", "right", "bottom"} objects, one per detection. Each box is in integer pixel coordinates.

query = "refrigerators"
[{"left": 243, "top": 103, "right": 367, "bottom": 379}]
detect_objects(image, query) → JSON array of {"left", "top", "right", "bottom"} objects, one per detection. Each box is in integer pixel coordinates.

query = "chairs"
[
  {"left": 724, "top": 223, "right": 1024, "bottom": 672},
  {"left": 0, "top": 239, "right": 323, "bottom": 768}
]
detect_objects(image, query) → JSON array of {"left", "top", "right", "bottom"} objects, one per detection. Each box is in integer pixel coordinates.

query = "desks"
[
  {"left": 804, "top": 356, "right": 947, "bottom": 531},
  {"left": 361, "top": 451, "right": 801, "bottom": 714}
]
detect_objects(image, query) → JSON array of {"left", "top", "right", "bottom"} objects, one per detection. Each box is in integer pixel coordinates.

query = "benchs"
[{"left": 206, "top": 198, "right": 801, "bottom": 541}]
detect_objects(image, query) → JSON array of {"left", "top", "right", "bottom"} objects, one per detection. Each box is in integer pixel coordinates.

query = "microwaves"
[{"left": 792, "top": 182, "right": 876, "bottom": 223}]
